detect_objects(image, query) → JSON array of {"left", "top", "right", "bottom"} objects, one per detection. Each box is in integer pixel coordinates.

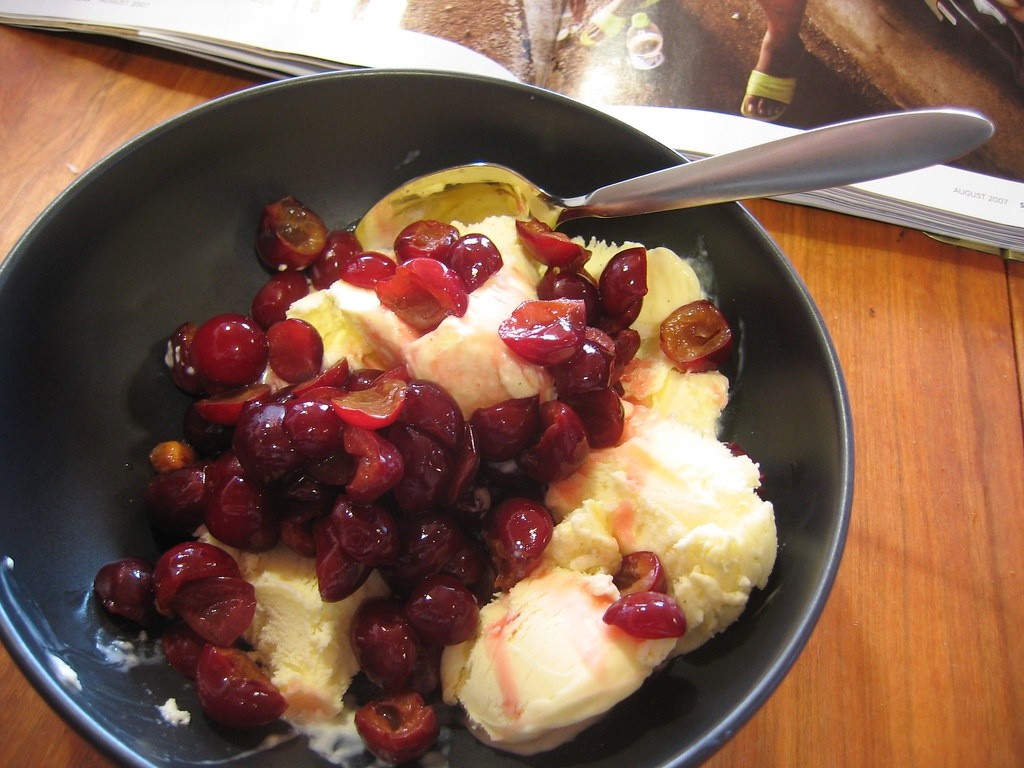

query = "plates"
[{"left": 0, "top": 66, "right": 855, "bottom": 768}]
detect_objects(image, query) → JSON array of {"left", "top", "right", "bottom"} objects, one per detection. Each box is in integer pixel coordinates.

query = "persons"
[{"left": 580, "top": 0, "right": 805, "bottom": 122}]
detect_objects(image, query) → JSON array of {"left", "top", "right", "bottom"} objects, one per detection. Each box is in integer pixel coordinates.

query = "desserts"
[{"left": 91, "top": 194, "right": 780, "bottom": 765}]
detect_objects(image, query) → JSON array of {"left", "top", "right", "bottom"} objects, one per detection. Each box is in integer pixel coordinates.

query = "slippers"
[
  {"left": 579, "top": 0, "right": 660, "bottom": 46},
  {"left": 741, "top": 49, "right": 804, "bottom": 122}
]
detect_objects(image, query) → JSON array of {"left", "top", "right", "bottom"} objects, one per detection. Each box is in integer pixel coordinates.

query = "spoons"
[{"left": 355, "top": 108, "right": 994, "bottom": 264}]
